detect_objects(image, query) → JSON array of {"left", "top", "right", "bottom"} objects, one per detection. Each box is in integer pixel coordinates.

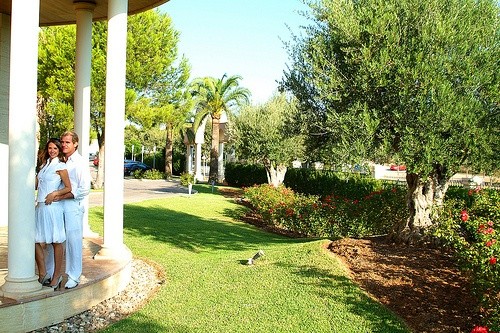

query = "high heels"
[
  {"left": 50, "top": 275, "right": 63, "bottom": 289},
  {"left": 42, "top": 273, "right": 52, "bottom": 285}
]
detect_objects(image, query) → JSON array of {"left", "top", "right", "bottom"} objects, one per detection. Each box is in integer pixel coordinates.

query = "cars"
[
  {"left": 125, "top": 161, "right": 152, "bottom": 177},
  {"left": 390, "top": 161, "right": 406, "bottom": 171},
  {"left": 352, "top": 165, "right": 369, "bottom": 176}
]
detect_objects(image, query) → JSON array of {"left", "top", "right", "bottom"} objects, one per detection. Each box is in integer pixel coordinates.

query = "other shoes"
[{"left": 64, "top": 279, "right": 77, "bottom": 288}]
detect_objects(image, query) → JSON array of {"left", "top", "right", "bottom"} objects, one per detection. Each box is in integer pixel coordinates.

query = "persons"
[
  {"left": 57, "top": 131, "right": 93, "bottom": 289},
  {"left": 35, "top": 137, "right": 72, "bottom": 289}
]
